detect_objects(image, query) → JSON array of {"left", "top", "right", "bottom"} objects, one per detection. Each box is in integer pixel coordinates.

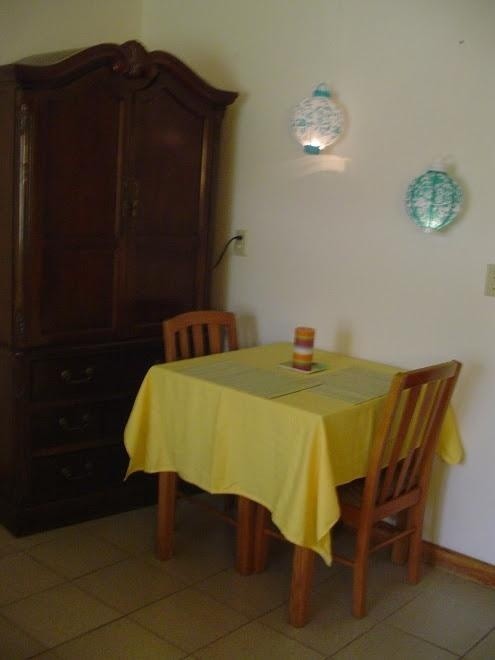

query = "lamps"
[
  {"left": 291, "top": 82, "right": 347, "bottom": 156},
  {"left": 404, "top": 154, "right": 465, "bottom": 236}
]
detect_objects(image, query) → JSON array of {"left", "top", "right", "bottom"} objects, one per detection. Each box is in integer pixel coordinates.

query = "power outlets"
[{"left": 234, "top": 230, "right": 248, "bottom": 256}]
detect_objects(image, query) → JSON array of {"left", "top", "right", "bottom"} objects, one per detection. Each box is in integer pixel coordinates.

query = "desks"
[{"left": 123, "top": 341, "right": 464, "bottom": 575}]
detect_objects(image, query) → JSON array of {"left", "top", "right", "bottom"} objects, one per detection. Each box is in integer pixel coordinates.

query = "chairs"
[
  {"left": 253, "top": 360, "right": 461, "bottom": 620},
  {"left": 162, "top": 312, "right": 254, "bottom": 574}
]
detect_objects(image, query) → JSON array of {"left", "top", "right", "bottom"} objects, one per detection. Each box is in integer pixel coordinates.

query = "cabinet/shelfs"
[{"left": 0, "top": 39, "right": 240, "bottom": 540}]
